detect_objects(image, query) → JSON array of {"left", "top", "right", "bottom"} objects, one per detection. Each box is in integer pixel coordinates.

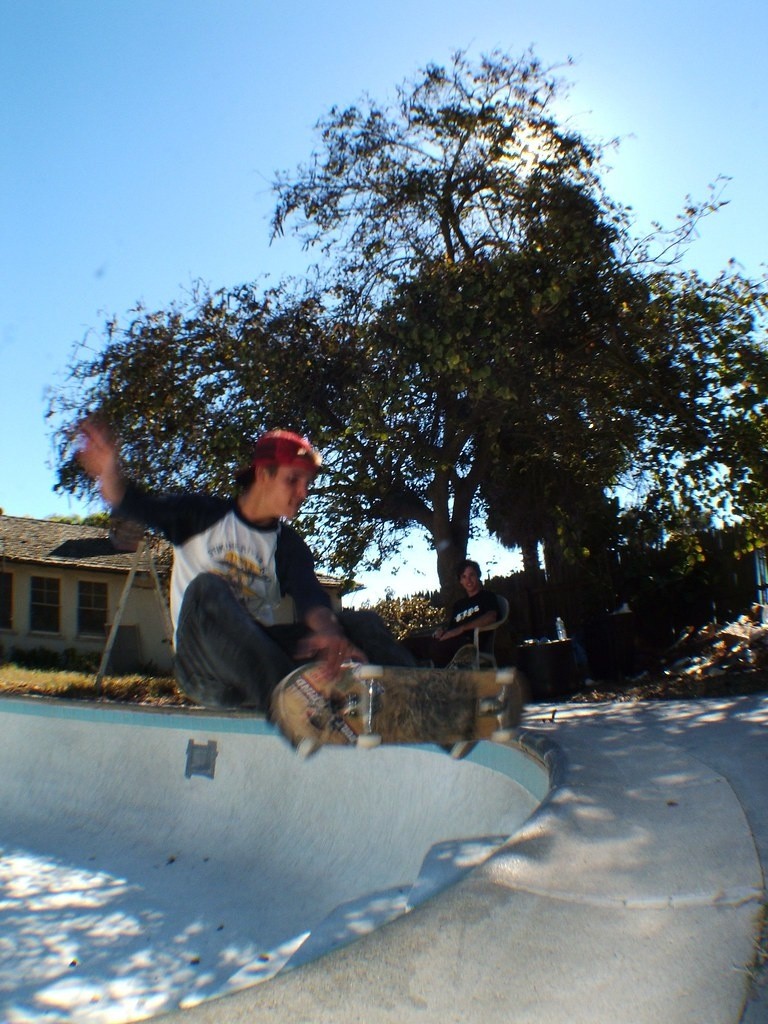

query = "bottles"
[{"left": 555, "top": 616, "right": 567, "bottom": 641}]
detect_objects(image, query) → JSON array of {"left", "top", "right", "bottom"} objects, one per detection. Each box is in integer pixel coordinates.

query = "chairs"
[{"left": 474, "top": 595, "right": 509, "bottom": 669}]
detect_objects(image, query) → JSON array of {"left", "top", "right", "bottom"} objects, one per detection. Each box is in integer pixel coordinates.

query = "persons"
[
  {"left": 434, "top": 561, "right": 502, "bottom": 672},
  {"left": 79, "top": 422, "right": 365, "bottom": 717}
]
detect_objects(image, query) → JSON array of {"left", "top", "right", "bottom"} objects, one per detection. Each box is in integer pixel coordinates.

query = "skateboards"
[{"left": 271, "top": 659, "right": 528, "bottom": 749}]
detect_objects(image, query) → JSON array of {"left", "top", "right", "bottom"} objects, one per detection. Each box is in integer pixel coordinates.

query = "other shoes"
[
  {"left": 438, "top": 737, "right": 478, "bottom": 760},
  {"left": 284, "top": 727, "right": 322, "bottom": 760}
]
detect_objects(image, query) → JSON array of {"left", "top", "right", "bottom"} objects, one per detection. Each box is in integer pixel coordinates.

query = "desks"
[{"left": 514, "top": 641, "right": 578, "bottom": 707}]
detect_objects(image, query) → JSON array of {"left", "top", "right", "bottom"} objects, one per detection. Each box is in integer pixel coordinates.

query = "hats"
[{"left": 251, "top": 430, "right": 323, "bottom": 472}]
